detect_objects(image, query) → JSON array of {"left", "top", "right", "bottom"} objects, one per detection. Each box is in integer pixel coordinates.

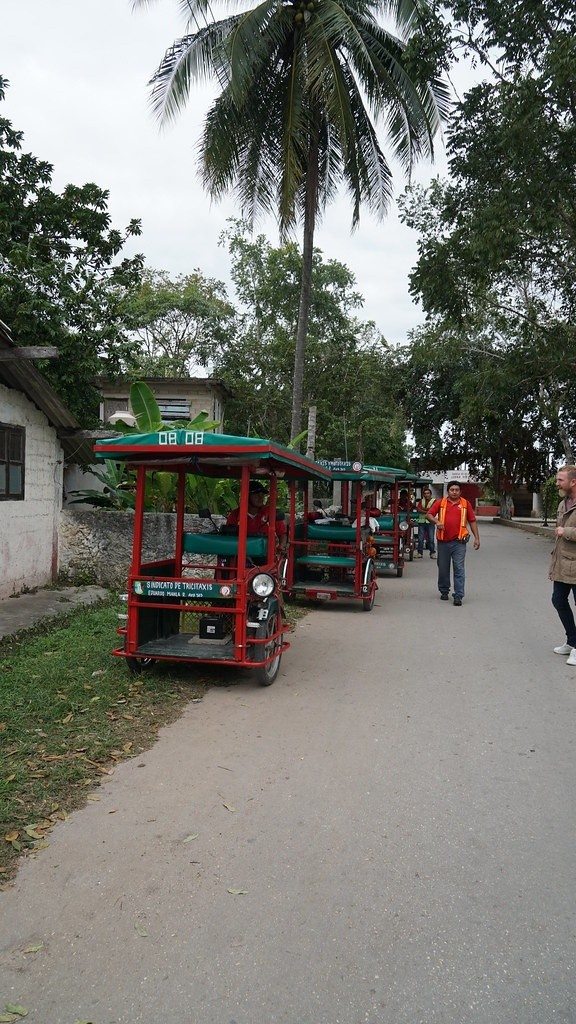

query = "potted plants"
[{"left": 476, "top": 485, "right": 514, "bottom": 516}]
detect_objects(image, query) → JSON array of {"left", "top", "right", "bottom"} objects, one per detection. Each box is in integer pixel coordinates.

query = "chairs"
[{"left": 218, "top": 524, "right": 256, "bottom": 581}]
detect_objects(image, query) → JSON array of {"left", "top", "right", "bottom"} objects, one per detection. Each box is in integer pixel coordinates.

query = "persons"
[
  {"left": 364, "top": 496, "right": 381, "bottom": 517},
  {"left": 425, "top": 481, "right": 480, "bottom": 606},
  {"left": 398, "top": 491, "right": 413, "bottom": 549},
  {"left": 413, "top": 489, "right": 437, "bottom": 558},
  {"left": 226, "top": 481, "right": 287, "bottom": 564},
  {"left": 547, "top": 465, "right": 576, "bottom": 665}
]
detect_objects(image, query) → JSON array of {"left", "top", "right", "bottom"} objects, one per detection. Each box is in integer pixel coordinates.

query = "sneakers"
[
  {"left": 430, "top": 553, "right": 438, "bottom": 559},
  {"left": 553, "top": 643, "right": 576, "bottom": 666},
  {"left": 454, "top": 597, "right": 462, "bottom": 606},
  {"left": 441, "top": 592, "right": 448, "bottom": 600},
  {"left": 414, "top": 554, "right": 423, "bottom": 558}
]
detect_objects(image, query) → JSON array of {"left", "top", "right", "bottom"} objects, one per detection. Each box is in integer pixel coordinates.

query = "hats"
[{"left": 248, "top": 481, "right": 269, "bottom": 494}]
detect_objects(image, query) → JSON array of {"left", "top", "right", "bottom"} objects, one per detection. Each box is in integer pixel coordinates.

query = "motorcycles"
[
  {"left": 91, "top": 428, "right": 332, "bottom": 687},
  {"left": 357, "top": 464, "right": 433, "bottom": 577},
  {"left": 276, "top": 457, "right": 379, "bottom": 613}
]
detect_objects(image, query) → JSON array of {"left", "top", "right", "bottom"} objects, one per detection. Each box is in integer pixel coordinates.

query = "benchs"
[
  {"left": 375, "top": 517, "right": 395, "bottom": 530},
  {"left": 307, "top": 525, "right": 357, "bottom": 540},
  {"left": 373, "top": 536, "right": 394, "bottom": 546},
  {"left": 184, "top": 533, "right": 267, "bottom": 557},
  {"left": 296, "top": 555, "right": 356, "bottom": 568}
]
